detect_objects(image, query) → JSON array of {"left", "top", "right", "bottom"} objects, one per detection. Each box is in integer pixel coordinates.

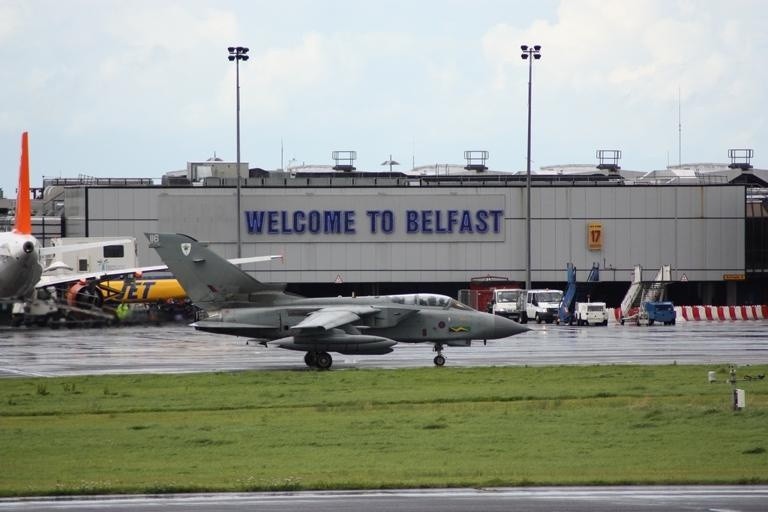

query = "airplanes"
[{"left": 142, "top": 231, "right": 534, "bottom": 370}]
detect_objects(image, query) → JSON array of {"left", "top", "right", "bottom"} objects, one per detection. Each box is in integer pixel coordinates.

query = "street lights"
[
  {"left": 518, "top": 45, "right": 542, "bottom": 292},
  {"left": 226, "top": 45, "right": 249, "bottom": 271}
]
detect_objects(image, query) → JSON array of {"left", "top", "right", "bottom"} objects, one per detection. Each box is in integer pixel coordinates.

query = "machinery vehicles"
[{"left": 469, "top": 262, "right": 677, "bottom": 325}]
